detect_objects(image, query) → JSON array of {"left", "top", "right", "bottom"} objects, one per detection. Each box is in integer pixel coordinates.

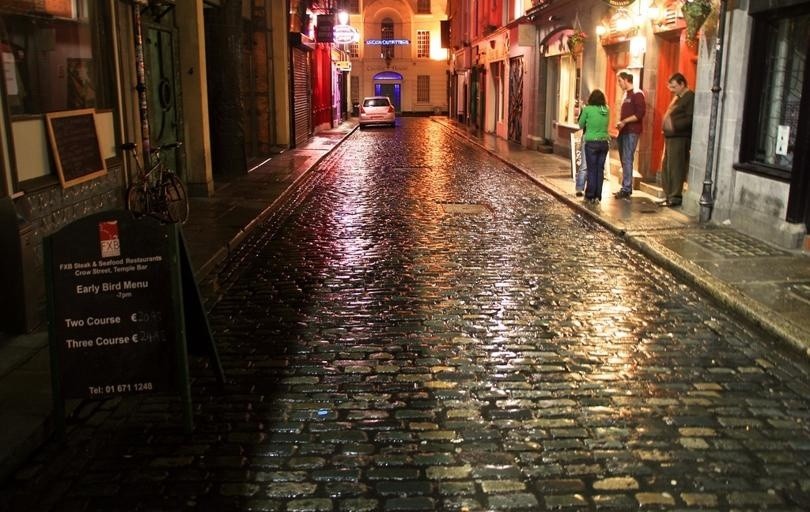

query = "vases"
[{"left": 569, "top": 46, "right": 585, "bottom": 60}]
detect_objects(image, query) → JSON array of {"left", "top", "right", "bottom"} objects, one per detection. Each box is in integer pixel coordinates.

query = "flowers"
[{"left": 567, "top": 32, "right": 588, "bottom": 51}]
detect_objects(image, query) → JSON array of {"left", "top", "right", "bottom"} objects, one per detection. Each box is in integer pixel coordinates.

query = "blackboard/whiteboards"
[{"left": 45, "top": 108, "right": 108, "bottom": 189}]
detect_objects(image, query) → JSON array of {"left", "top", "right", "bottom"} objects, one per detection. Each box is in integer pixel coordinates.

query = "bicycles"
[{"left": 122, "top": 142, "right": 189, "bottom": 227}]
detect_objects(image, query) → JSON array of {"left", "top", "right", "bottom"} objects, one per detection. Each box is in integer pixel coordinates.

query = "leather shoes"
[
  {"left": 615, "top": 191, "right": 632, "bottom": 199},
  {"left": 659, "top": 201, "right": 679, "bottom": 207}
]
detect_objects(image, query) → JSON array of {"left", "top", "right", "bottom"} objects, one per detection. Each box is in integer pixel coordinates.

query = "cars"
[{"left": 359, "top": 96, "right": 396, "bottom": 129}]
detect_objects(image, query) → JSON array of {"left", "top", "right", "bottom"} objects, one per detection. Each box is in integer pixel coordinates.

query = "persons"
[
  {"left": 575, "top": 103, "right": 588, "bottom": 197},
  {"left": 578, "top": 89, "right": 610, "bottom": 204},
  {"left": 655, "top": 73, "right": 695, "bottom": 206},
  {"left": 612, "top": 72, "right": 647, "bottom": 200}
]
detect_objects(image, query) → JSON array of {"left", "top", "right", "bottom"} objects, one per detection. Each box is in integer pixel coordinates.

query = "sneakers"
[{"left": 576, "top": 191, "right": 599, "bottom": 204}]
